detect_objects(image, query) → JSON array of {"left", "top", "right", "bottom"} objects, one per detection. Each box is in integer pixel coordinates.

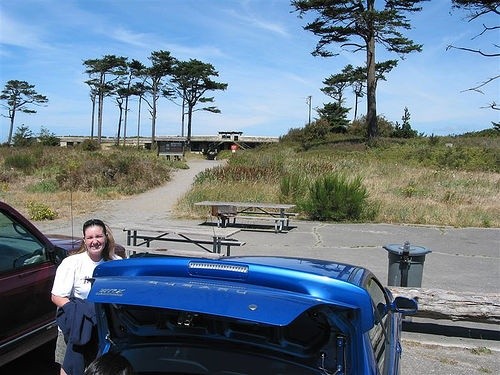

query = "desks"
[
  {"left": 193, "top": 201, "right": 296, "bottom": 230},
  {"left": 122, "top": 224, "right": 241, "bottom": 254}
]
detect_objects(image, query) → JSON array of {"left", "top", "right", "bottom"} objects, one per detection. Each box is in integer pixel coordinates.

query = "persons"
[
  {"left": 51, "top": 219, "right": 123, "bottom": 375},
  {"left": 84, "top": 353, "right": 134, "bottom": 375}
]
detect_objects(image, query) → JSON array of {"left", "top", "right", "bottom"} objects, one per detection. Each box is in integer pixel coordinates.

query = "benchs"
[
  {"left": 124, "top": 234, "right": 246, "bottom": 259},
  {"left": 217, "top": 210, "right": 299, "bottom": 233}
]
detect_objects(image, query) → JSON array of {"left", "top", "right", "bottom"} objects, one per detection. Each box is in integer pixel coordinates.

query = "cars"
[
  {"left": 1, "top": 199, "right": 126, "bottom": 370},
  {"left": 86, "top": 254, "right": 419, "bottom": 373}
]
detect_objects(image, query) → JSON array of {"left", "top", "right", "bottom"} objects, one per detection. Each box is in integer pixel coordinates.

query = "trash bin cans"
[{"left": 382, "top": 241, "right": 432, "bottom": 288}]
